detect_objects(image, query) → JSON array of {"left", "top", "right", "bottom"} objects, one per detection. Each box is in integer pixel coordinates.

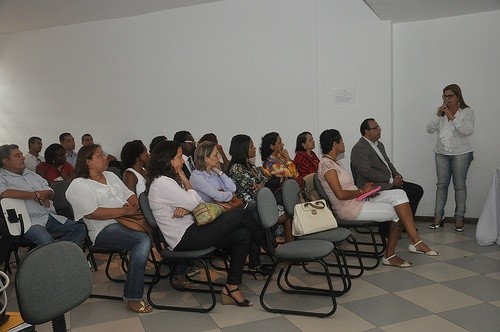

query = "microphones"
[{"left": 441, "top": 99, "right": 451, "bottom": 116}]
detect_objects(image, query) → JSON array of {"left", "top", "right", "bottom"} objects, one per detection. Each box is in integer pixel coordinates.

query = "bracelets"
[
  {"left": 359, "top": 189, "right": 362, "bottom": 194},
  {"left": 219, "top": 171, "right": 223, "bottom": 176}
]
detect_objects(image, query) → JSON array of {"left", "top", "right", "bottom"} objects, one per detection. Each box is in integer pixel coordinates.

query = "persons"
[
  {"left": 174, "top": 131, "right": 196, "bottom": 180},
  {"left": 351, "top": 118, "right": 424, "bottom": 239},
  {"left": 199, "top": 134, "right": 229, "bottom": 172},
  {"left": 65, "top": 144, "right": 192, "bottom": 314},
  {"left": 35, "top": 143, "right": 74, "bottom": 185},
  {"left": 82, "top": 134, "right": 117, "bottom": 161},
  {"left": 60, "top": 133, "right": 77, "bottom": 169},
  {"left": 189, "top": 141, "right": 271, "bottom": 280},
  {"left": 426, "top": 84, "right": 475, "bottom": 232},
  {"left": 318, "top": 130, "right": 440, "bottom": 267},
  {"left": 24, "top": 137, "right": 46, "bottom": 173},
  {"left": 0, "top": 144, "right": 87, "bottom": 249},
  {"left": 121, "top": 136, "right": 201, "bottom": 277},
  {"left": 227, "top": 135, "right": 294, "bottom": 244},
  {"left": 293, "top": 132, "right": 320, "bottom": 194},
  {"left": 144, "top": 141, "right": 278, "bottom": 307},
  {"left": 261, "top": 132, "right": 298, "bottom": 185}
]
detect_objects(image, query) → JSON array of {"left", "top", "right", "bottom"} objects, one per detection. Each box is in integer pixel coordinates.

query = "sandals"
[
  {"left": 409, "top": 239, "right": 441, "bottom": 256},
  {"left": 123, "top": 295, "right": 152, "bottom": 312},
  {"left": 172, "top": 275, "right": 193, "bottom": 289}
]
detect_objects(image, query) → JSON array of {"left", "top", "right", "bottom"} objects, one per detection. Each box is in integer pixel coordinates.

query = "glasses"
[
  {"left": 369, "top": 126, "right": 380, "bottom": 129},
  {"left": 442, "top": 94, "right": 456, "bottom": 98},
  {"left": 382, "top": 253, "right": 413, "bottom": 267}
]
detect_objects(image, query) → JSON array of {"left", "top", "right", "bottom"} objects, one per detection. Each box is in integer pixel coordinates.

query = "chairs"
[{"left": 0, "top": 168, "right": 390, "bottom": 332}]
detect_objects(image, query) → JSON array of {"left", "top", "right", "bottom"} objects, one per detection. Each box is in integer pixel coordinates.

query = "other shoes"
[{"left": 252, "top": 265, "right": 272, "bottom": 276}]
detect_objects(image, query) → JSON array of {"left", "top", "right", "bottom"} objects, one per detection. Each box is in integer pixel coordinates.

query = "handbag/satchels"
[
  {"left": 116, "top": 202, "right": 153, "bottom": 233},
  {"left": 214, "top": 189, "right": 243, "bottom": 211},
  {"left": 291, "top": 191, "right": 338, "bottom": 237},
  {"left": 193, "top": 202, "right": 224, "bottom": 225}
]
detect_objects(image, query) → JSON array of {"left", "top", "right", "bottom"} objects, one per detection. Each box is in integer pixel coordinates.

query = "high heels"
[
  {"left": 429, "top": 215, "right": 444, "bottom": 228},
  {"left": 221, "top": 284, "right": 253, "bottom": 306},
  {"left": 455, "top": 218, "right": 464, "bottom": 231}
]
